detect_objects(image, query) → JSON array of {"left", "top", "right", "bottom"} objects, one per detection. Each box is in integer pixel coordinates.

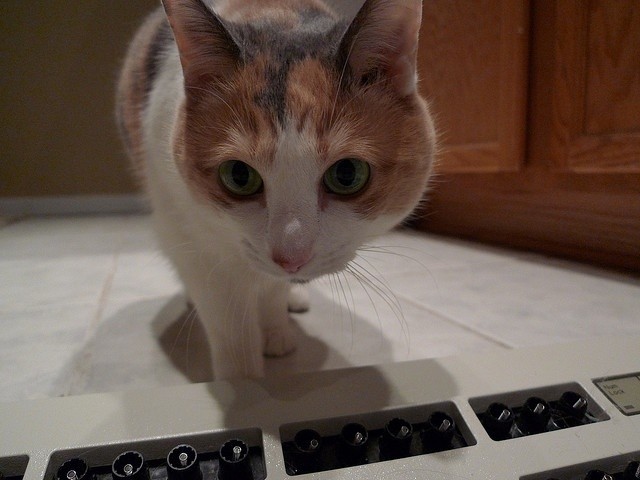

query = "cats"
[{"left": 114, "top": 0, "right": 452, "bottom": 414}]
